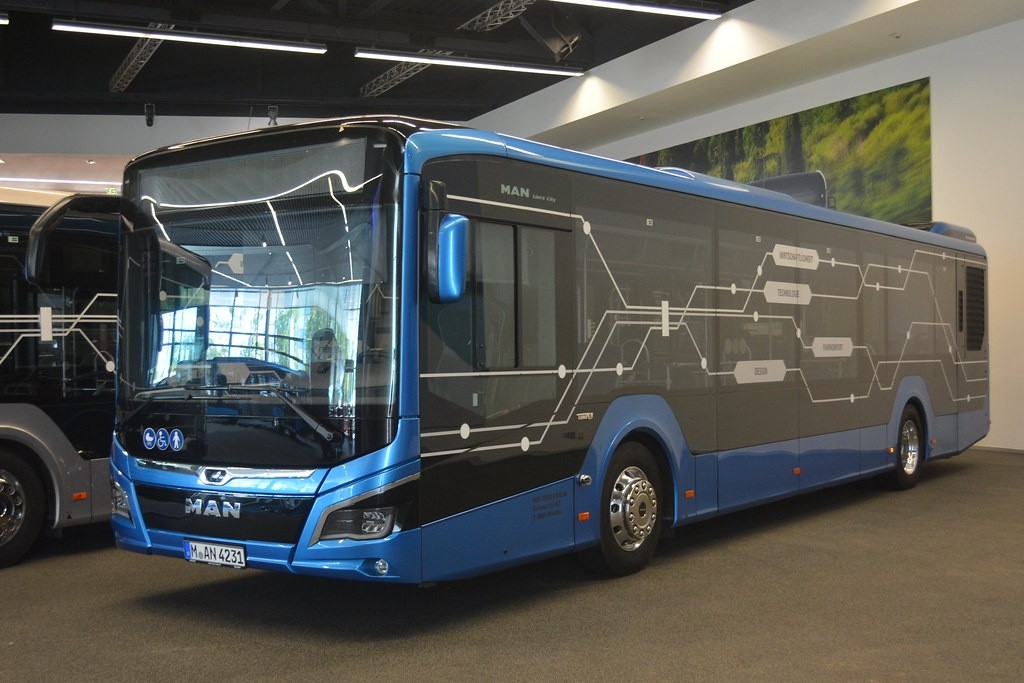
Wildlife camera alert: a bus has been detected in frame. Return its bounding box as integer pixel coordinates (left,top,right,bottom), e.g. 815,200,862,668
24,115,993,586
0,187,122,566
138,242,212,460
741,172,832,208
